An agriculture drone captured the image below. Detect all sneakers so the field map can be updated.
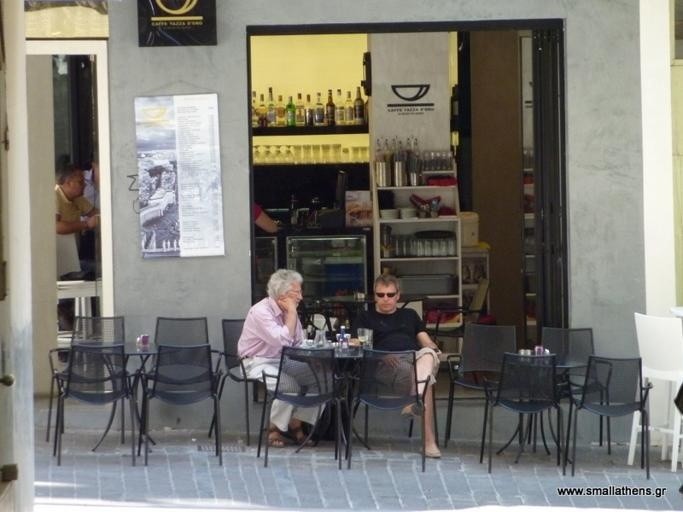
[420,446,441,458]
[401,402,426,421]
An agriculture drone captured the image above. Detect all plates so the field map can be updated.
[380,207,417,221]
[427,177,458,186]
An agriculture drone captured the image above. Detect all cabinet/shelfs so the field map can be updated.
[369,157,461,362]
[250,124,368,294]
[462,243,491,322]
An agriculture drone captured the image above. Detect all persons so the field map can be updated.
[252,199,278,235]
[53,162,100,282]
[345,273,444,459]
[78,160,99,261]
[237,267,329,448]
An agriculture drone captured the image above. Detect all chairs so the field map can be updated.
[424,278,491,358]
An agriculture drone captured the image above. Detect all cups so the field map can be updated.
[253,144,368,164]
[357,328,371,351]
[391,237,456,257]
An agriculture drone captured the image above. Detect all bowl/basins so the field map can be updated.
[301,333,365,348]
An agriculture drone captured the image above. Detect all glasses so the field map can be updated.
[375,293,397,298]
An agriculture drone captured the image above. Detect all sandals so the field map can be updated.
[282,424,312,445]
[266,427,285,447]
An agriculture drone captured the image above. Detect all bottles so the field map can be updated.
[372,137,423,189]
[421,151,454,171]
[251,85,364,127]
[336,325,349,357]
[287,199,299,224]
[313,329,328,349]
